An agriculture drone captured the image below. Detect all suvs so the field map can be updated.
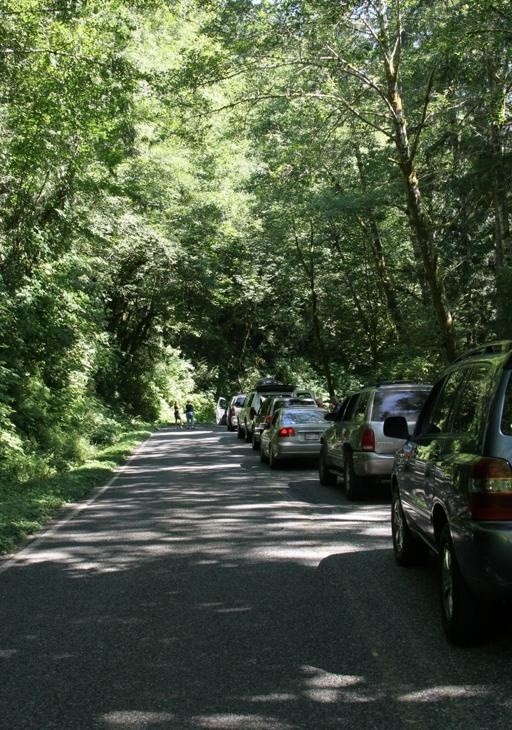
[382,339,511,649]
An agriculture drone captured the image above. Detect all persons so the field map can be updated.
[186,399,195,429]
[174,401,182,430]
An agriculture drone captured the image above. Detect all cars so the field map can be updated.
[250,397,319,451]
[258,405,336,471]
[318,379,434,502]
[215,384,316,444]
